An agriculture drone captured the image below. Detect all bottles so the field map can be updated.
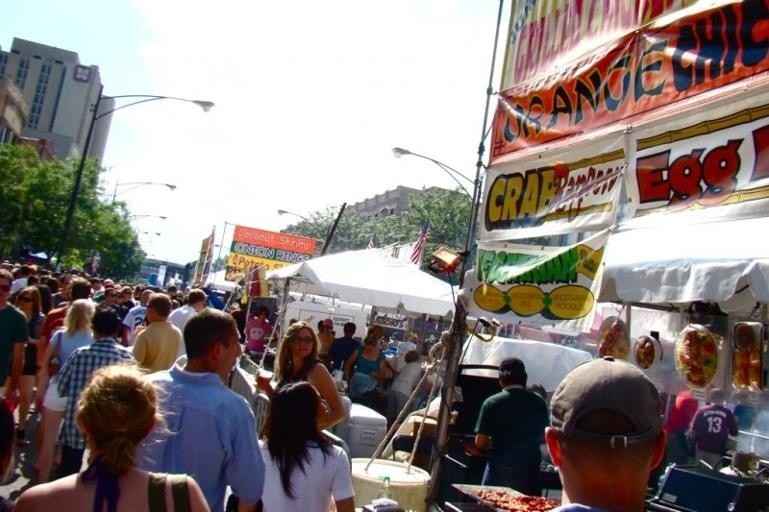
[378,477,390,500]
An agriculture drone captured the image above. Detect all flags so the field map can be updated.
[407,217,431,264]
[364,234,379,252]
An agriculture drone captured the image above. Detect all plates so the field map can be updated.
[597,316,719,390]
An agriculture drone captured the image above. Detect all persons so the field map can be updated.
[660,385,767,469]
[540,358,668,511]
[1,258,451,512]
[470,356,550,495]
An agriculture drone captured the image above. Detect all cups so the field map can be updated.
[255,370,273,393]
[335,370,343,382]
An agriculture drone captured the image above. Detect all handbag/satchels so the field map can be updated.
[225,491,264,512]
[47,331,62,374]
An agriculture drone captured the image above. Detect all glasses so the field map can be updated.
[16,296,34,303]
[292,334,314,344]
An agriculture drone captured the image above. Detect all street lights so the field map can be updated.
[392,144,483,289]
[57,87,217,281]
[276,209,333,253]
[111,179,177,238]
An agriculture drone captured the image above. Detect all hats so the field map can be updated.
[547,354,664,448]
[322,318,334,329]
